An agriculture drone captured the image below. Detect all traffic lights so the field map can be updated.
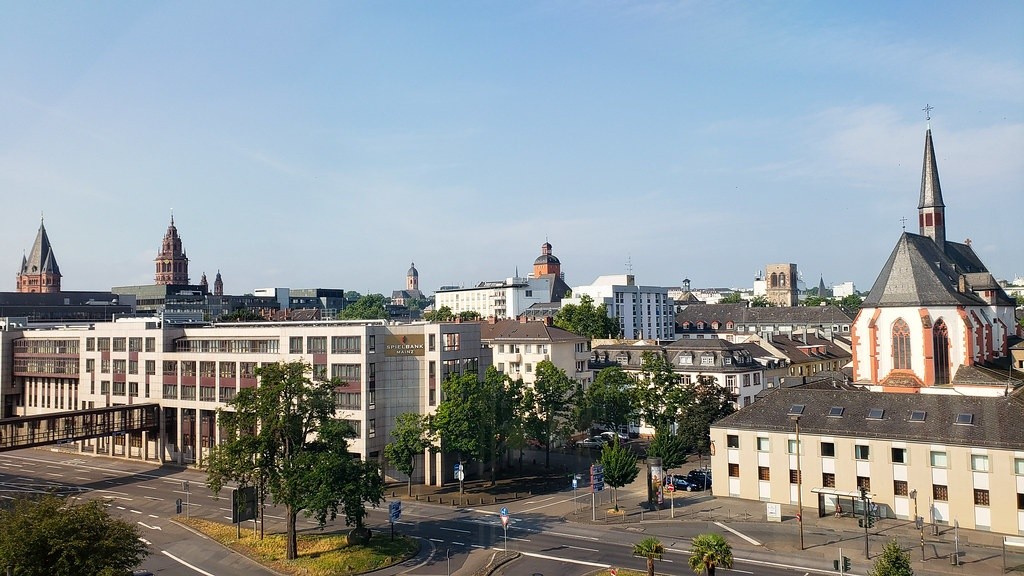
[860,487,867,500]
[833,560,839,571]
[843,557,851,572]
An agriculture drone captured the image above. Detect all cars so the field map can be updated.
[687,469,712,489]
[575,431,630,450]
[663,475,703,492]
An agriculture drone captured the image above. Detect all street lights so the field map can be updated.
[791,415,803,550]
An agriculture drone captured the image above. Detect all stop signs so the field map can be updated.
[796,514,800,520]
[667,483,674,492]
